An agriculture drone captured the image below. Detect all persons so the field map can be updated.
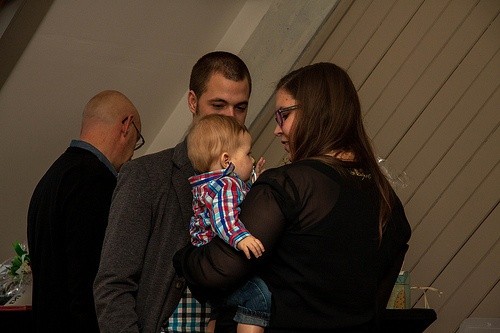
[172,62,411,332]
[28,89,145,333]
[94,52,261,333]
[188,113,269,333]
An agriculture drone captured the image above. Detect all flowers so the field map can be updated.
[0,241,31,287]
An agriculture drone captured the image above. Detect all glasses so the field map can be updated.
[122,117,145,150]
[275,105,299,128]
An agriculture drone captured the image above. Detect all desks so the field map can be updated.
[0,303,438,333]
